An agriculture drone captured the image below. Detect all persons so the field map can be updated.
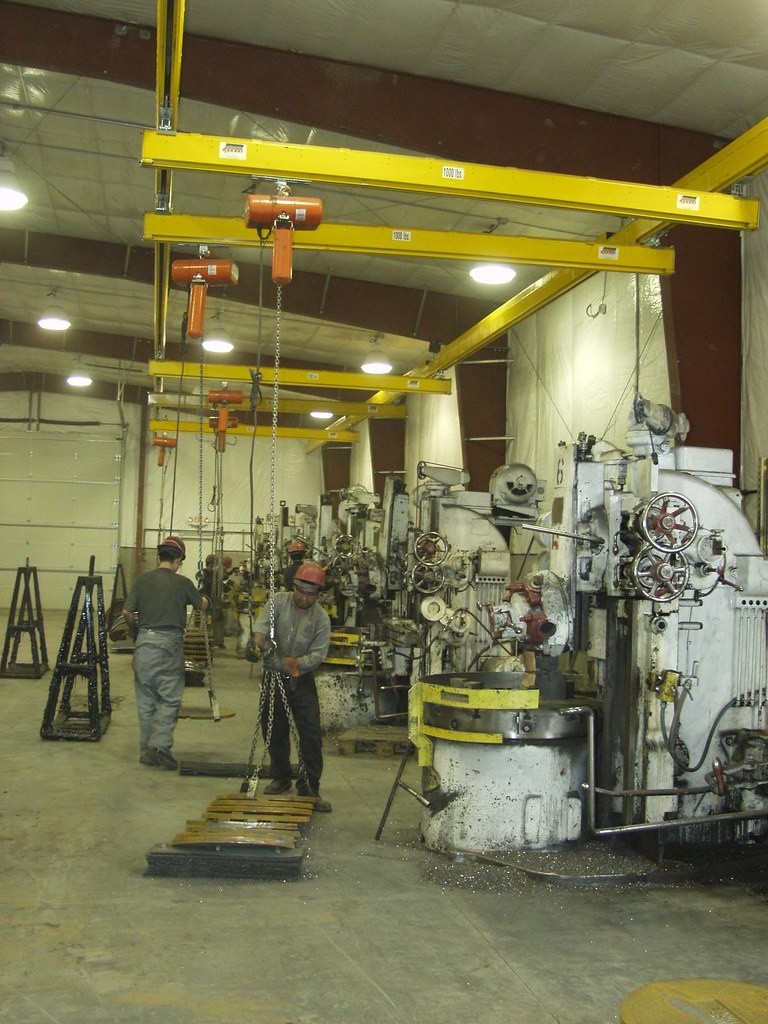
[244,562,331,812]
[283,540,308,592]
[122,535,209,771]
[221,556,237,636]
[202,553,212,597]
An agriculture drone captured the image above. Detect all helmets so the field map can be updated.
[156,536,187,562]
[205,554,215,563]
[287,541,307,553]
[223,557,232,565]
[292,563,325,590]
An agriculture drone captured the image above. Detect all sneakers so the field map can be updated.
[263,778,293,795]
[140,749,158,766]
[145,746,178,770]
[298,790,332,811]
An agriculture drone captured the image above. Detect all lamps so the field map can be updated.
[66,368,92,386]
[361,350,393,374]
[0,155,29,211]
[37,306,71,331]
[203,328,235,352]
